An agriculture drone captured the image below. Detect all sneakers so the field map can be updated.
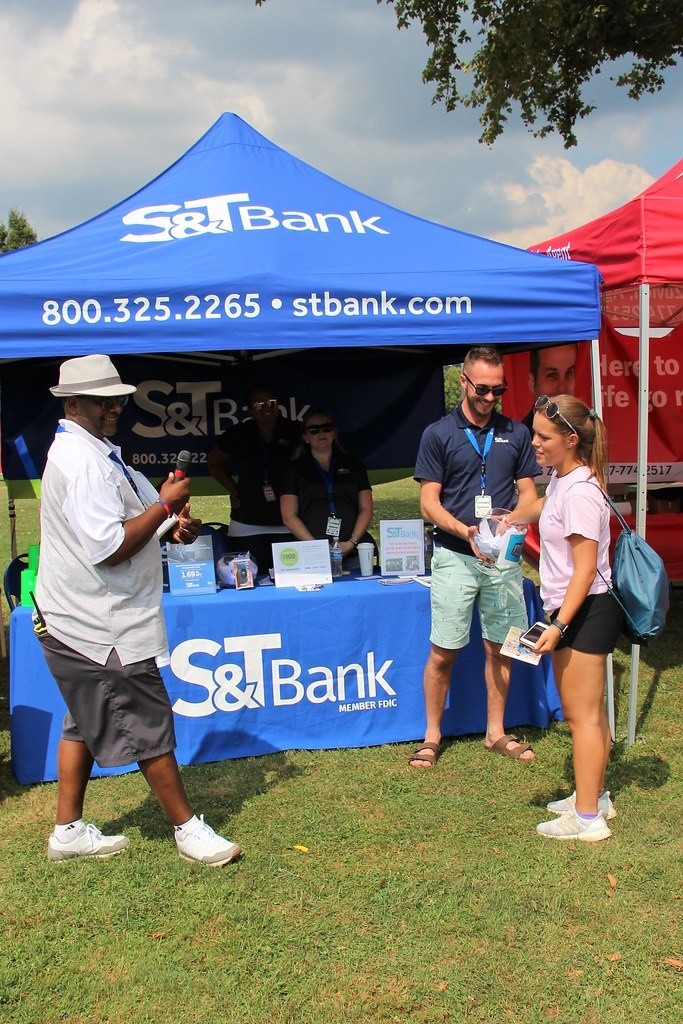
[536,803,612,842]
[175,814,242,866]
[48,824,130,864]
[547,789,616,820]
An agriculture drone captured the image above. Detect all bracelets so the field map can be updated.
[550,624,565,638]
[155,499,170,514]
[350,536,359,546]
[503,515,511,528]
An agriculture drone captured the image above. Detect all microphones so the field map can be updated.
[173,450,191,483]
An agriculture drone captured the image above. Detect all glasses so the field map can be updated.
[253,399,277,410]
[463,373,508,396]
[305,423,333,435]
[75,395,128,411]
[535,395,577,434]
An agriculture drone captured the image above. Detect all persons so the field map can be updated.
[209,378,309,575]
[280,408,379,571]
[407,348,544,767]
[32,353,241,867]
[520,340,578,442]
[494,394,626,841]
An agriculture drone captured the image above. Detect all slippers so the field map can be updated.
[408,742,440,768]
[484,733,535,761]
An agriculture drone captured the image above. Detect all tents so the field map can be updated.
[499,156,683,746]
[2,109,616,745]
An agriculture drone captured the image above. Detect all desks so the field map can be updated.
[8,582,563,784]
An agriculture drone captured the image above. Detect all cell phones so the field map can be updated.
[519,622,550,648]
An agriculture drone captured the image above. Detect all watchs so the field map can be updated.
[551,619,569,634]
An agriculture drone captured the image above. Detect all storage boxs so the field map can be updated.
[165,531,224,595]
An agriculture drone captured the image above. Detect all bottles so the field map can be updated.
[330,537,343,577]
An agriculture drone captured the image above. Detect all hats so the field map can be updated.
[50,354,137,396]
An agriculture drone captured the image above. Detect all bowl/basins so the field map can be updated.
[474,508,527,576]
[217,552,258,586]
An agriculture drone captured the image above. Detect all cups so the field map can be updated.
[357,543,375,576]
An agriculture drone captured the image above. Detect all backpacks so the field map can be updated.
[563,481,669,647]
